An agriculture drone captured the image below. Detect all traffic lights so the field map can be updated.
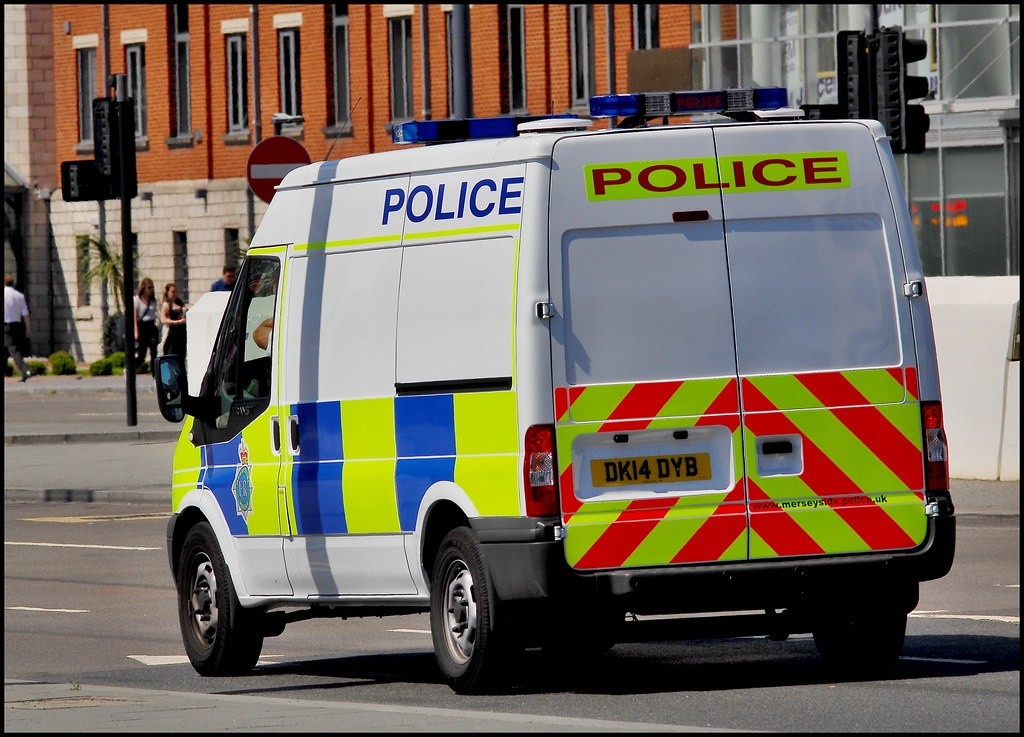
[90,98,134,177]
[866,24,931,158]
[799,103,841,120]
[836,29,874,121]
[60,159,138,201]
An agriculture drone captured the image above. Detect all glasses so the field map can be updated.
[145,286,154,289]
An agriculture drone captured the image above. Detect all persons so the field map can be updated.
[160,283,190,360]
[123,277,162,379]
[211,264,238,291]
[4,273,33,382]
[253,317,273,357]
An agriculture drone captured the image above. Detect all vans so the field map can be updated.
[151,85,958,696]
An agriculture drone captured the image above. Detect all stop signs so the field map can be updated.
[246,136,311,205]
